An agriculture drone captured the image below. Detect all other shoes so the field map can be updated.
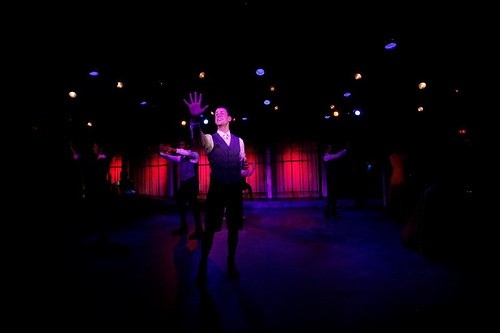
[197,259,207,273]
[227,254,240,277]
[172,227,188,236]
[188,230,203,240]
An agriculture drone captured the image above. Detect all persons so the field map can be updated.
[182,91,249,279]
[69,141,108,200]
[145,139,203,240]
[321,144,347,220]
